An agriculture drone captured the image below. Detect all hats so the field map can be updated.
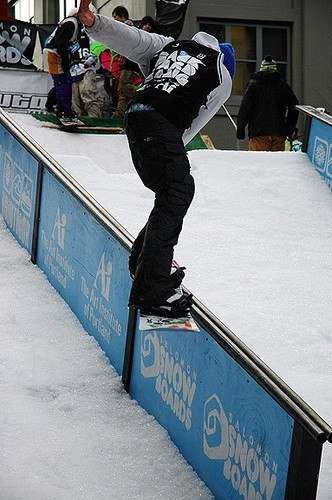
[219,43,235,81]
[259,55,277,72]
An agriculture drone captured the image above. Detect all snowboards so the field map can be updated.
[137,259,201,333]
[41,107,85,127]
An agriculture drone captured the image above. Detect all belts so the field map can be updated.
[125,103,155,114]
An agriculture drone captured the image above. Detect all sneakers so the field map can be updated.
[129,266,185,289]
[140,292,194,318]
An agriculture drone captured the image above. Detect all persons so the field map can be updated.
[235,56,299,151]
[76,7,234,319]
[285,123,303,151]
[43,3,153,128]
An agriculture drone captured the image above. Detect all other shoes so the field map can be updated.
[63,117,80,122]
